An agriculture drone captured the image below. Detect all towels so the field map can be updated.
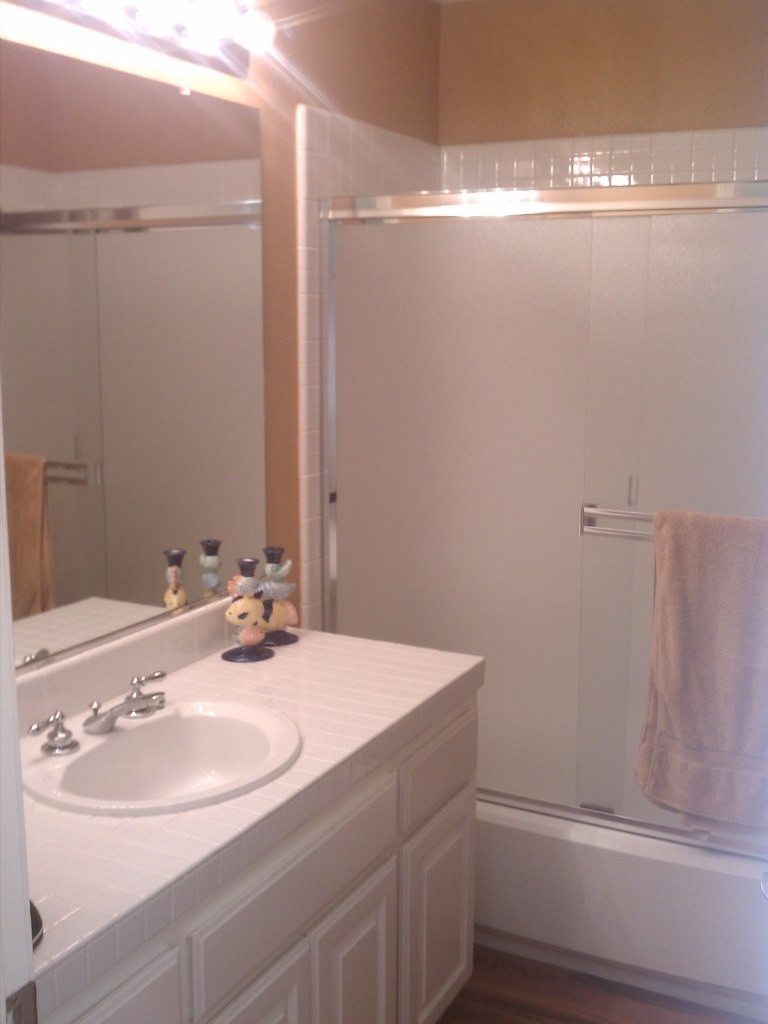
[5,449,58,622]
[632,505,768,834]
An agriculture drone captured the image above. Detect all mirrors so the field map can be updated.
[0,37,268,668]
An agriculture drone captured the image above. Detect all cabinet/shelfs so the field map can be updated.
[72,693,478,1024]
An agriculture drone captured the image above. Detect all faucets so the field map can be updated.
[83,692,166,736]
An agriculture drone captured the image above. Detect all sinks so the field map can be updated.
[21,698,300,818]
